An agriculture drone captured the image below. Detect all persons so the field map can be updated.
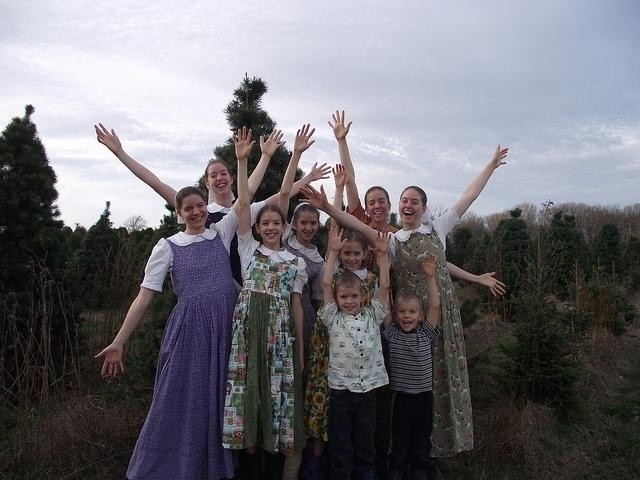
[317,227,388,479]
[222,128,308,480]
[94,124,332,290]
[303,164,388,480]
[278,124,345,480]
[298,144,509,479]
[376,255,441,479]
[328,110,401,233]
[94,131,288,480]
